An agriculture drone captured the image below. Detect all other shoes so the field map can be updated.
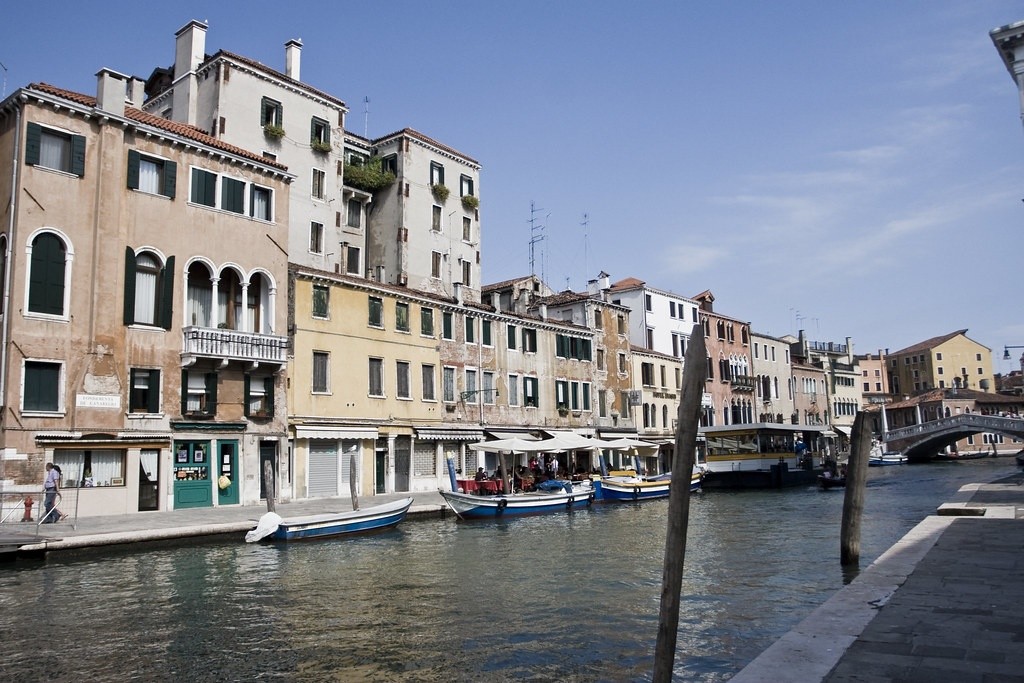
[42,521,52,523]
[54,515,61,523]
[60,514,68,520]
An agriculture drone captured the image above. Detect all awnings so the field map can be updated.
[545,431,583,437]
[33,429,171,443]
[415,429,486,441]
[295,424,379,440]
[489,432,538,440]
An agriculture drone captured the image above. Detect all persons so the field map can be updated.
[454,455,615,495]
[41,462,62,524]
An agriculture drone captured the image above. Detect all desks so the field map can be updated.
[456,478,511,496]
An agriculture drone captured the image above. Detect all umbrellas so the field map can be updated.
[468,437,657,494]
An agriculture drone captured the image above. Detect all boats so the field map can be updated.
[439,489,597,519]
[601,472,702,501]
[699,425,838,490]
[818,474,847,487]
[275,496,414,538]
[869,453,908,465]
